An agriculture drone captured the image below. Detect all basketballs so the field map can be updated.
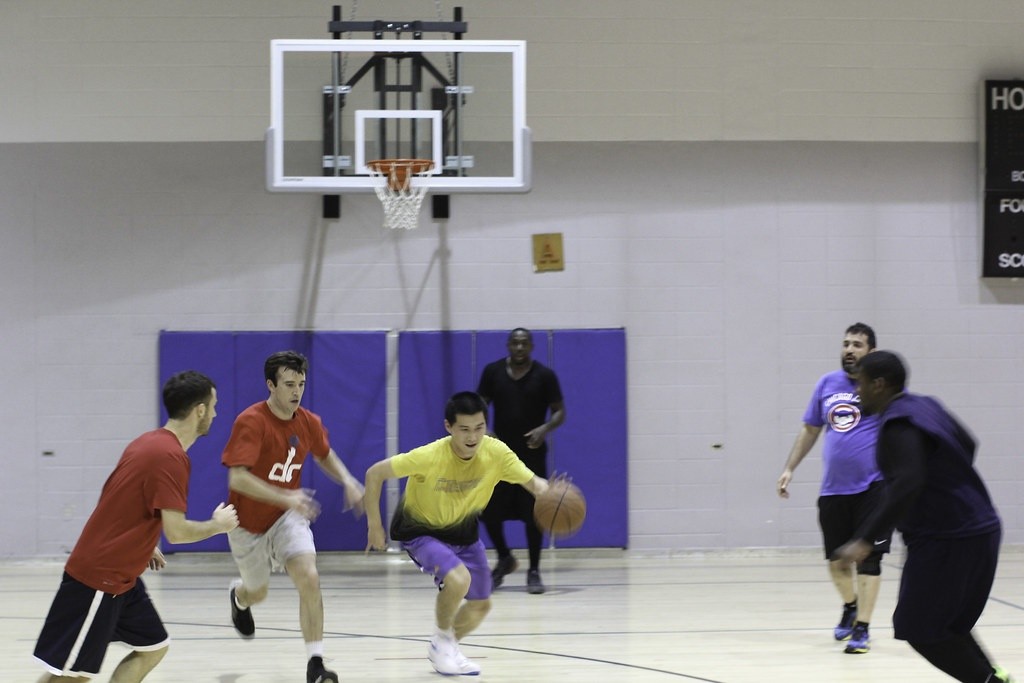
[530,482,586,536]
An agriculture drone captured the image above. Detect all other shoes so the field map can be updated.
[991,665,1016,683]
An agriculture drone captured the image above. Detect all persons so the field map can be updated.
[34,370,241,683]
[775,324,895,653]
[834,351,1011,683]
[364,390,570,676]
[222,351,362,683]
[477,328,566,594]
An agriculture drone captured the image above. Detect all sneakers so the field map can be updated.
[834,598,857,641]
[228,579,256,639]
[844,624,872,652]
[490,553,517,589]
[527,568,546,594]
[427,635,460,675]
[454,642,482,675]
[306,655,339,683]
